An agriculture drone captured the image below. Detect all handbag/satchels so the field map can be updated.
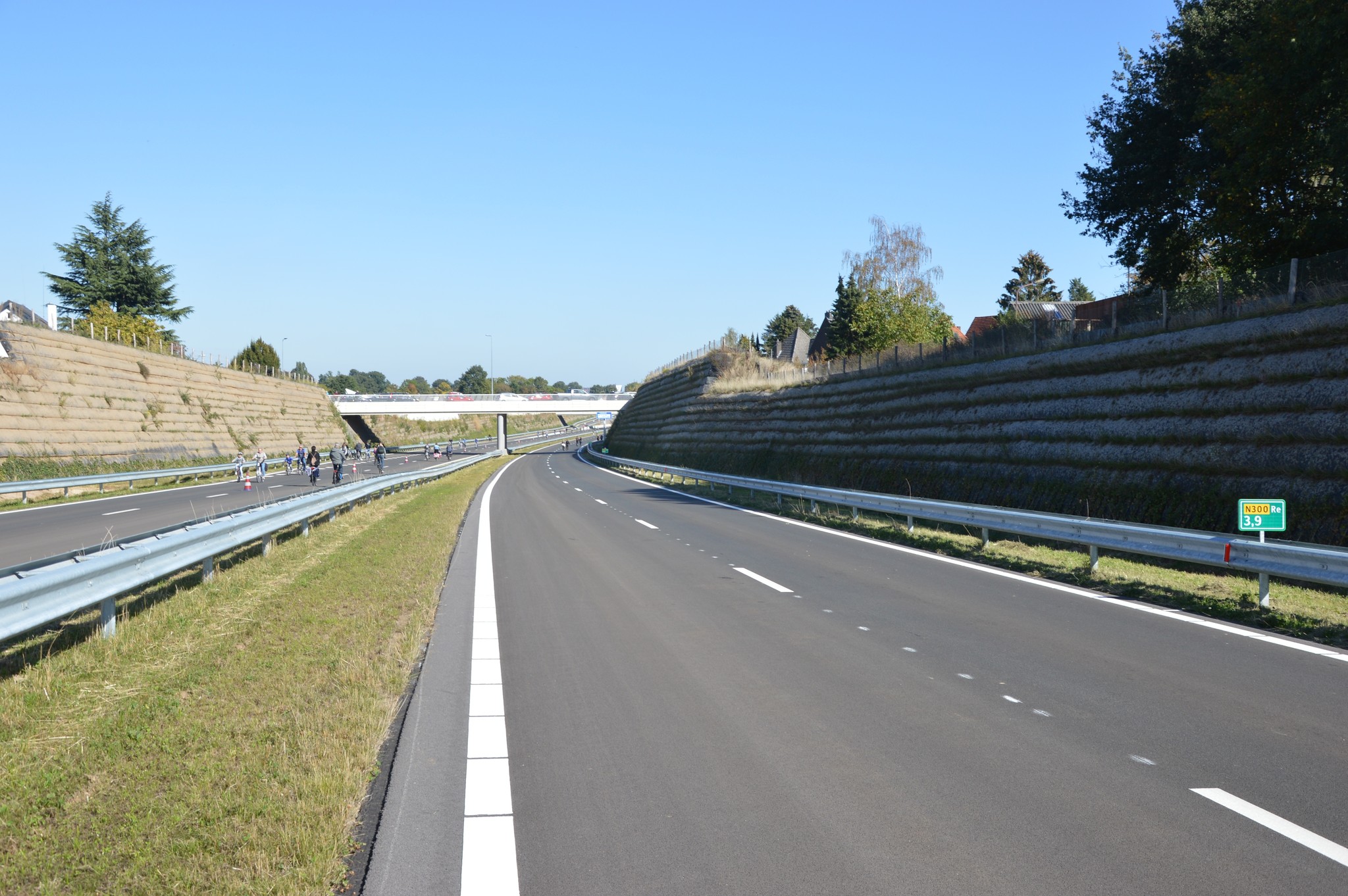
[265,463,268,471]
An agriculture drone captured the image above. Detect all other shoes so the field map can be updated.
[332,482,335,484]
[263,477,265,479]
[381,470,384,472]
[235,472,237,475]
[241,476,243,478]
[291,470,293,472]
[378,464,379,466]
[563,449,565,450]
[342,476,344,479]
[302,469,305,472]
[310,480,312,482]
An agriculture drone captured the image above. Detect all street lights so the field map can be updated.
[486,335,500,403]
[1015,279,1047,318]
[281,337,288,376]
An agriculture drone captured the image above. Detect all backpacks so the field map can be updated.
[311,452,317,466]
[378,446,384,454]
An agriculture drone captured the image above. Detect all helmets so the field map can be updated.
[238,452,242,455]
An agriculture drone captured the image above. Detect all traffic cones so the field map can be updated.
[243,472,254,492]
[484,444,487,448]
[405,455,409,463]
[352,461,359,474]
[464,447,468,453]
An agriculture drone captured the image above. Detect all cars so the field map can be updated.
[328,388,422,401]
[447,393,474,401]
[496,388,637,401]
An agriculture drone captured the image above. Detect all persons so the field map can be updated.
[562,439,566,451]
[294,446,307,472]
[446,444,453,458]
[433,443,441,454]
[231,452,245,479]
[592,429,603,441]
[575,436,579,446]
[565,439,570,451]
[537,424,588,439]
[251,447,267,479]
[424,443,431,459]
[284,454,294,471]
[448,437,453,446]
[489,434,493,441]
[330,439,387,480]
[458,438,462,446]
[307,446,320,483]
[462,438,466,448]
[579,437,582,446]
[475,438,478,446]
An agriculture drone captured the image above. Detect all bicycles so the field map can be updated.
[459,442,466,449]
[253,457,267,483]
[435,452,439,462]
[309,466,318,486]
[296,456,305,475]
[364,448,370,462]
[447,452,453,461]
[342,447,365,460]
[374,454,382,474]
[234,461,248,485]
[426,452,429,462]
[333,464,343,485]
[284,460,294,476]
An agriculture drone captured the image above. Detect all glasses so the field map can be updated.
[299,447,302,448]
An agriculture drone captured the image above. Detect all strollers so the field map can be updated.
[475,442,479,448]
